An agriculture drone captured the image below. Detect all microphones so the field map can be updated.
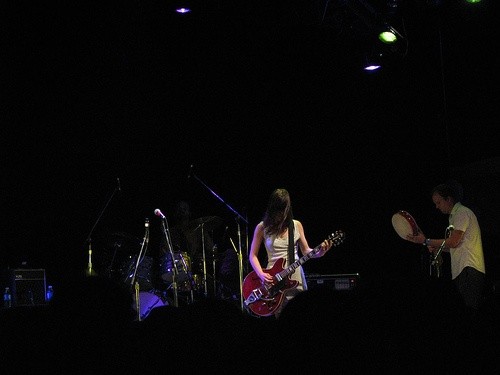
[185,164,194,180]
[117,178,122,190]
[445,224,456,233]
[154,208,166,219]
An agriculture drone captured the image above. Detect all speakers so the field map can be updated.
[12,268,47,306]
[304,272,361,293]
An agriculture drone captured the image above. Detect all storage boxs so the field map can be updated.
[9,269,47,307]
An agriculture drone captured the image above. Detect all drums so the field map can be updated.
[131,289,170,323]
[127,255,162,291]
[392,209,418,240]
[158,250,192,284]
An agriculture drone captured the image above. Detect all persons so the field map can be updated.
[160,201,214,258]
[407,184,486,294]
[248,190,333,316]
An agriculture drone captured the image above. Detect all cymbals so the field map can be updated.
[183,215,223,240]
[96,231,140,257]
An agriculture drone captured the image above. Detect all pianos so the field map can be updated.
[305,272,361,291]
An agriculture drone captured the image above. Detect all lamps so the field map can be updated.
[378,22,398,43]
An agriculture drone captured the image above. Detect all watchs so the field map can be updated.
[423,239,431,246]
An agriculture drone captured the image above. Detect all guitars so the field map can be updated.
[242,230,346,317]
